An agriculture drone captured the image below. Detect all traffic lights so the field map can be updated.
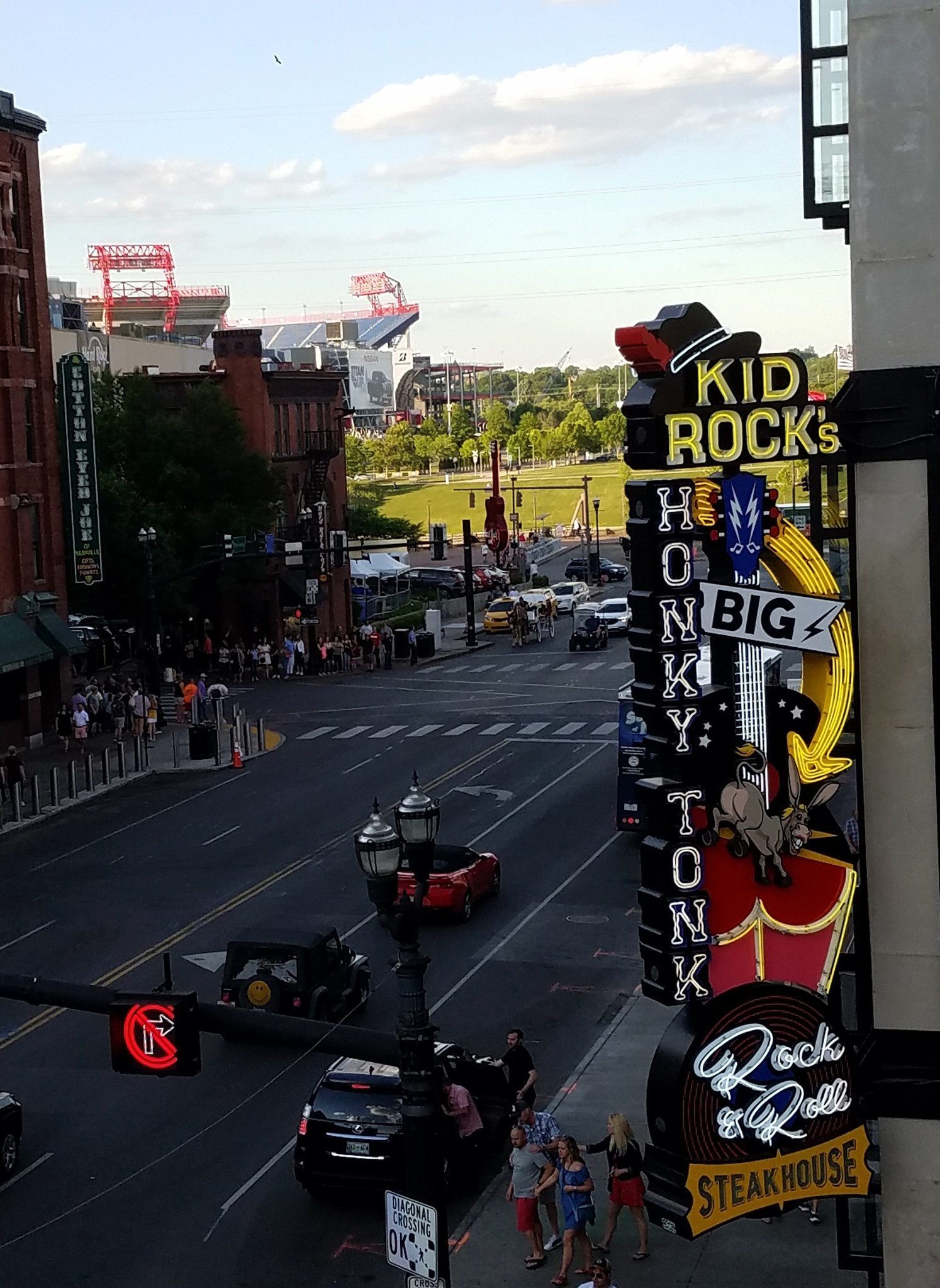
[295,608,305,619]
[516,491,523,508]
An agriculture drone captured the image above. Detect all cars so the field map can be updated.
[597,597,633,635]
[550,581,591,616]
[482,595,519,634]
[0,1091,23,1172]
[439,564,513,593]
[377,841,502,924]
[516,588,560,619]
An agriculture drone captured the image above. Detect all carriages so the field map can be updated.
[511,599,556,648]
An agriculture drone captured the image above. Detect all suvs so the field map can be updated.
[293,1043,507,1191]
[380,566,466,600]
[563,556,629,582]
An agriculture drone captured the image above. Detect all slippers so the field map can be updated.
[524,1254,548,1270]
[632,1251,651,1261]
[550,1274,568,1285]
[592,1244,610,1254]
[575,1266,590,1274]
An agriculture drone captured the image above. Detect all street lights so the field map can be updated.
[355,774,443,1285]
[511,477,518,558]
[138,527,158,623]
[593,499,604,587]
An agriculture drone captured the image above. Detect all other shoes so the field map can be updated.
[319,670,342,676]
[155,730,162,734]
[272,671,303,680]
[252,677,259,682]
[113,738,124,744]
[148,737,157,741]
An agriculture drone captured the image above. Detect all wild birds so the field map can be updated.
[274,54,282,65]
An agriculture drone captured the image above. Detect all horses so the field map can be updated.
[504,610,530,648]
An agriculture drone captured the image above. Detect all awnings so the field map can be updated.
[0,606,89,674]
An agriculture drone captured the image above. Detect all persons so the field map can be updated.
[56,670,229,754]
[184,615,393,684]
[506,1108,562,1270]
[577,1113,650,1261]
[534,1135,595,1287]
[439,1076,484,1196]
[519,597,531,612]
[530,561,538,575]
[486,1028,538,1125]
[408,625,420,668]
[494,586,502,600]
[0,745,26,806]
[578,1257,617,1288]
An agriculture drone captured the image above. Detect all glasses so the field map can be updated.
[610,1114,616,1119]
[512,1124,522,1130]
[590,1272,603,1279]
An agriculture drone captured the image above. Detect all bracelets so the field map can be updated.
[573,1186,577,1193]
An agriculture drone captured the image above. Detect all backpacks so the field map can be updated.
[211,688,221,699]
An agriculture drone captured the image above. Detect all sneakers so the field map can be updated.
[544,1234,563,1251]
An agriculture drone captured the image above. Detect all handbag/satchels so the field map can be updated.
[574,1204,596,1221]
[366,669,374,673]
[260,657,266,663]
[344,664,362,672]
[285,651,293,658]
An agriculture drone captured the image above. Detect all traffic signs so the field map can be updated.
[299,618,321,626]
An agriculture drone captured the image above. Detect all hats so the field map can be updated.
[509,1100,528,1117]
[8,745,16,753]
[200,673,208,677]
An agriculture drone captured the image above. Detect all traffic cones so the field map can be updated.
[229,739,246,769]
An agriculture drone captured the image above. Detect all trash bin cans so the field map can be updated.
[416,631,436,658]
[394,628,410,659]
[190,722,219,760]
